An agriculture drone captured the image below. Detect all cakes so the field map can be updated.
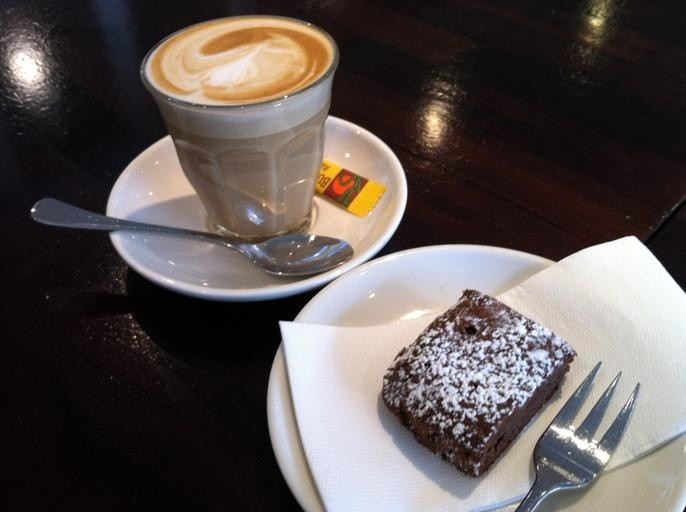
[382,288,576,478]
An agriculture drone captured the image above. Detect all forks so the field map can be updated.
[515,358,642,512]
[265,243,685,512]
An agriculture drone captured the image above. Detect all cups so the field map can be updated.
[141,13,341,245]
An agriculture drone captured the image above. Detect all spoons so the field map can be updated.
[29,196,355,278]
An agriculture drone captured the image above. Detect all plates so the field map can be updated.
[106,114,410,304]
